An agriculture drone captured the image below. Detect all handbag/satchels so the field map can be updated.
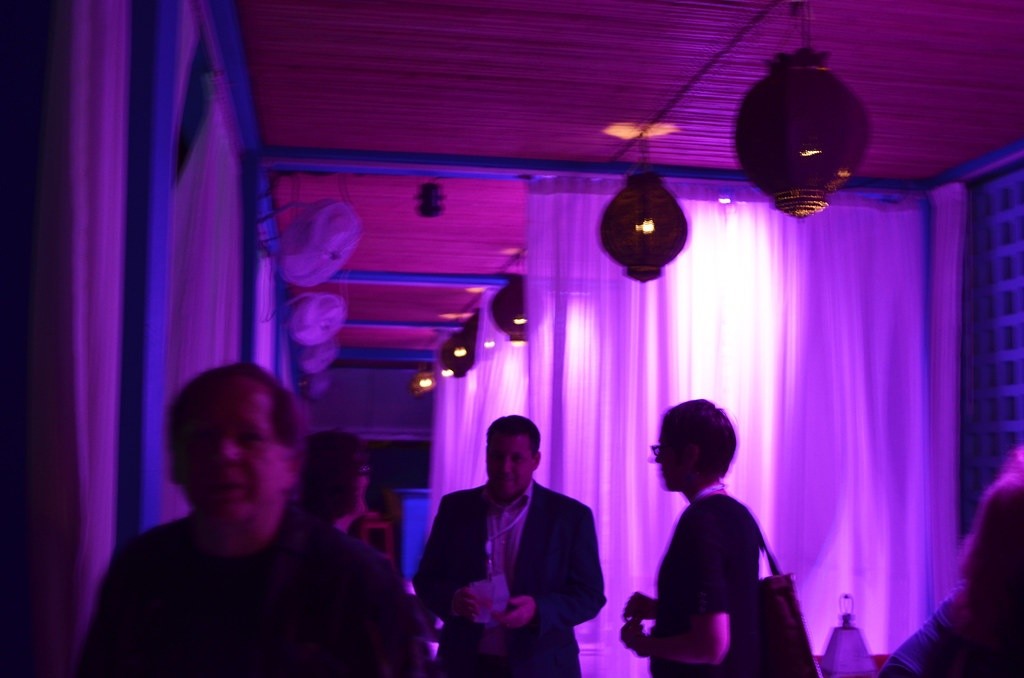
[741,500,823,678]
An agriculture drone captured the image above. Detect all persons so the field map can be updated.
[70,360,429,678]
[875,445,1024,678]
[411,414,608,678]
[619,398,774,678]
[295,429,380,532]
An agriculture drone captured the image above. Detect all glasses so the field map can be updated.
[359,465,369,474]
[652,444,667,457]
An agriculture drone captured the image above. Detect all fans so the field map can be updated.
[248,199,362,397]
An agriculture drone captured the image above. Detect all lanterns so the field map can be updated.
[438,330,475,377]
[411,369,437,399]
[595,171,687,282]
[488,282,528,342]
[728,48,868,220]
